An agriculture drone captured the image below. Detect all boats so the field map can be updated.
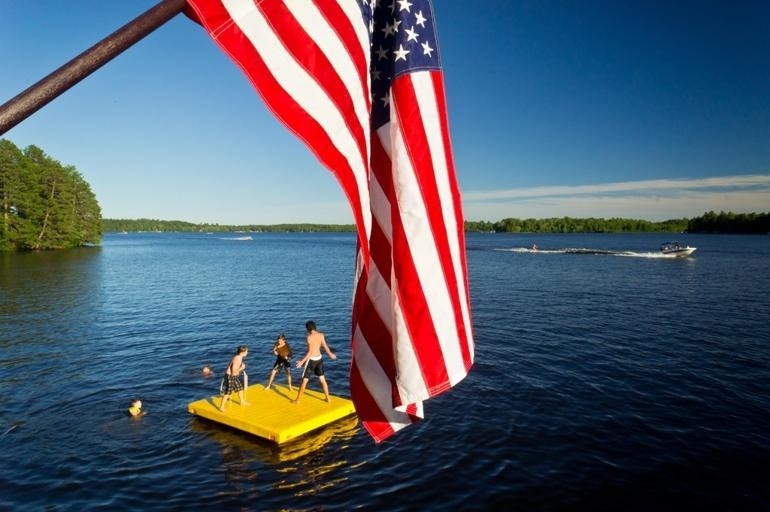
[661,242,697,258]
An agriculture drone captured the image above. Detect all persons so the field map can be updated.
[130,400,148,418]
[289,320,337,405]
[201,365,216,380]
[220,345,251,413]
[264,334,294,392]
[533,244,538,252]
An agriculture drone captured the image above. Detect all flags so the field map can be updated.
[178,0,476,445]
